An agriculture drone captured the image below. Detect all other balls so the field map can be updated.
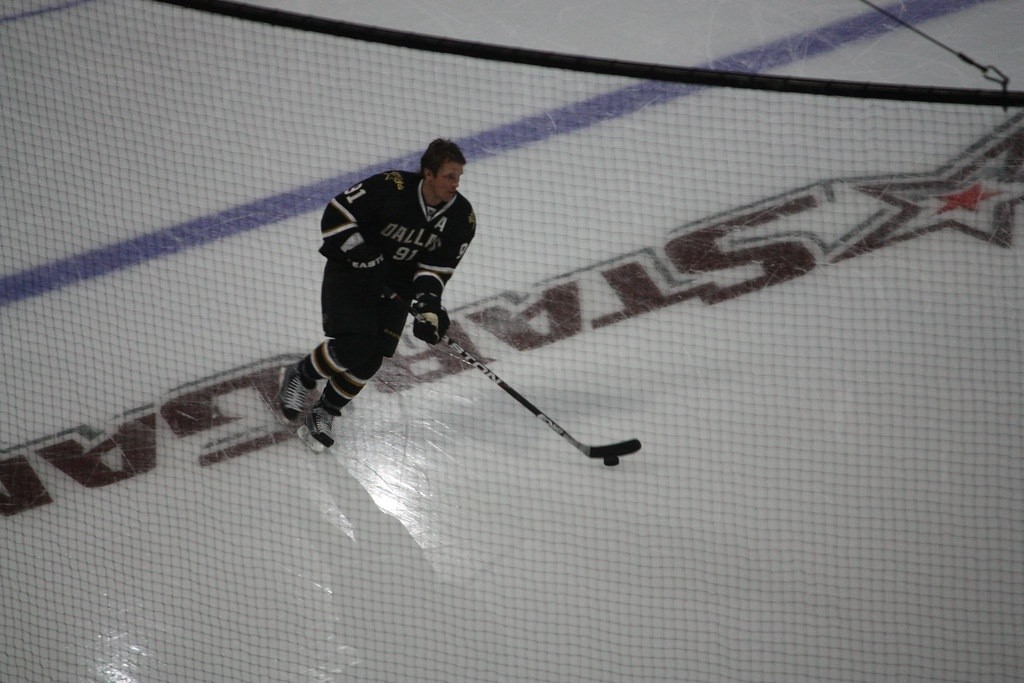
[603,456,619,466]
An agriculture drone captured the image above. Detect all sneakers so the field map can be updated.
[279,364,315,424]
[297,398,341,451]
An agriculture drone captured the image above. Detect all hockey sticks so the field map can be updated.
[384,289,644,461]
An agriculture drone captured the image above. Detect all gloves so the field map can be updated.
[412,293,451,345]
[343,241,393,287]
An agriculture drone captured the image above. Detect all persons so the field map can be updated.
[278,139,476,453]
